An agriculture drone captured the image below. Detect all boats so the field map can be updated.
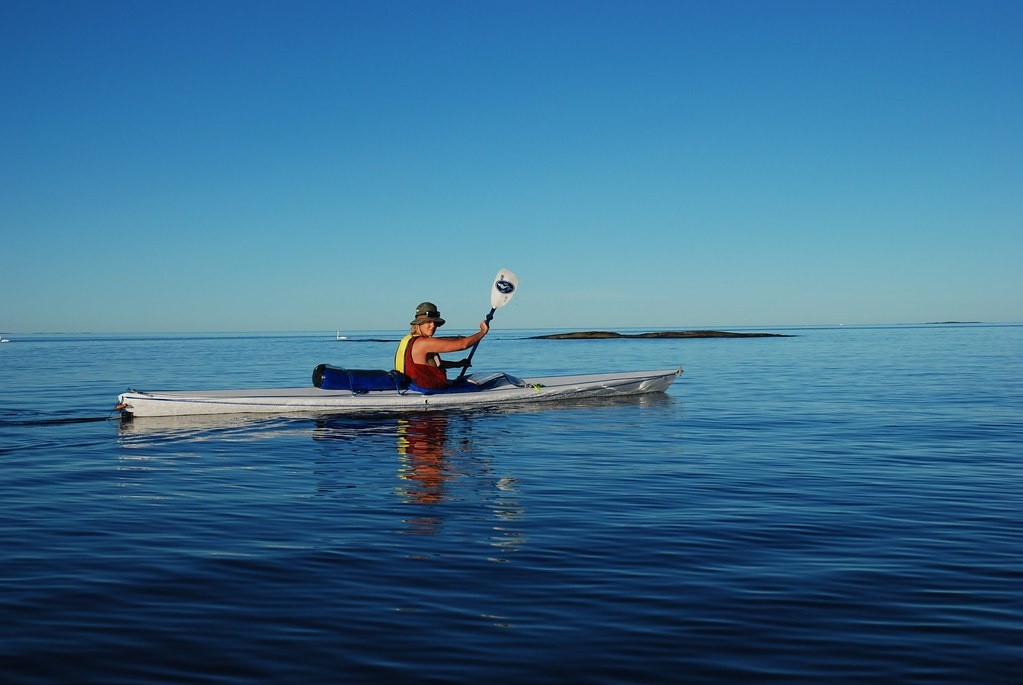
[116,366,685,418]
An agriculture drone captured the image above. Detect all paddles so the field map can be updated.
[459,268,518,377]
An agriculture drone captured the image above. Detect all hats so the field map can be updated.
[410,302,445,327]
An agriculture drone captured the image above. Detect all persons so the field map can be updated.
[395,302,489,394]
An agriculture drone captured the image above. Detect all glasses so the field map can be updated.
[415,311,440,318]
